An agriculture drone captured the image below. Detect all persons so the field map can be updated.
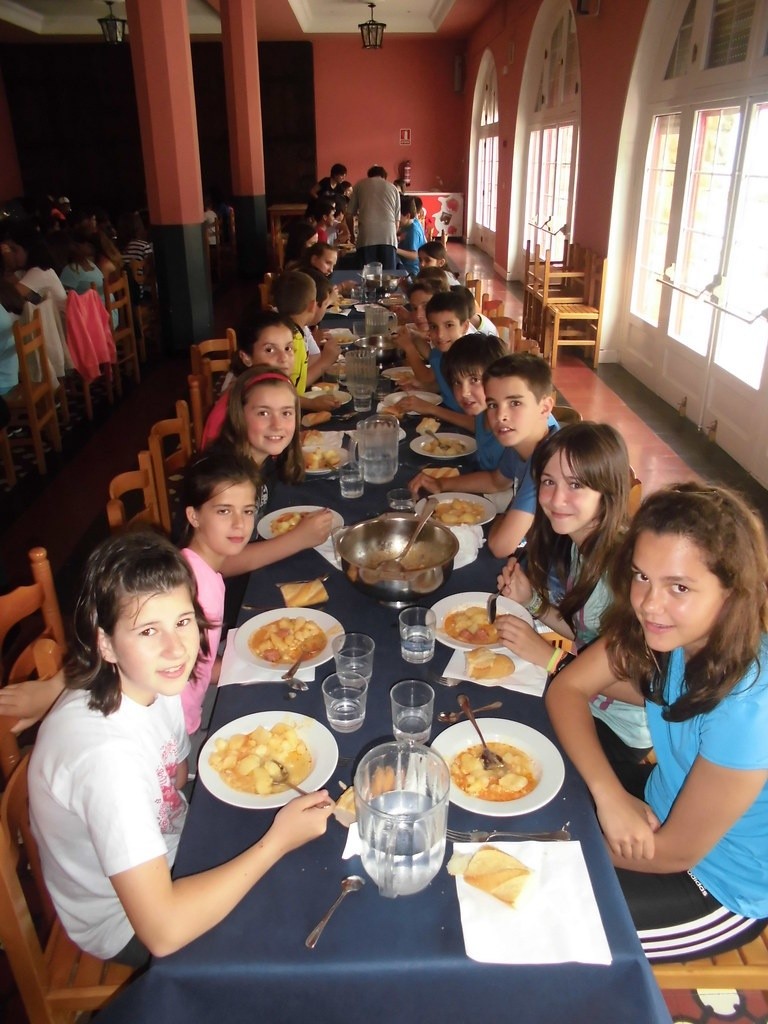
[0,452,261,775]
[283,194,359,292]
[0,196,158,436]
[409,352,560,557]
[204,198,232,249]
[496,420,656,765]
[28,539,334,969]
[390,249,561,472]
[201,267,340,465]
[547,481,767,964]
[311,163,428,271]
[218,364,335,636]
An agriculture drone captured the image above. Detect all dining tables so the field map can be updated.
[95,269,668,1024]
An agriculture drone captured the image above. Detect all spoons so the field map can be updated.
[281,636,323,680]
[425,429,452,450]
[436,701,503,723]
[487,549,526,626]
[458,695,504,770]
[263,760,331,810]
[305,875,365,949]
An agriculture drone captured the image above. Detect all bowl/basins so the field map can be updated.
[353,336,405,364]
[335,516,460,607]
[366,273,401,291]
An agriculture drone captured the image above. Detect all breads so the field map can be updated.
[280,578,329,607]
[303,430,325,445]
[338,784,368,815]
[422,467,459,478]
[463,845,530,907]
[381,406,405,418]
[416,418,441,435]
[463,647,515,681]
[331,305,339,313]
[302,411,332,427]
[313,382,340,390]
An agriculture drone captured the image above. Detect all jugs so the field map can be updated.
[336,346,380,399]
[348,414,399,485]
[364,306,398,336]
[353,739,451,899]
[364,261,382,288]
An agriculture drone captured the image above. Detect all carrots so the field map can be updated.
[211,721,309,795]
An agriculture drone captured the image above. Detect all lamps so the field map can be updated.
[358,3,386,48]
[98,1,126,44]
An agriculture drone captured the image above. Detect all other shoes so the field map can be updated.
[6,425,22,437]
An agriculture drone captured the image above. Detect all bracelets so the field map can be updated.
[341,282,344,290]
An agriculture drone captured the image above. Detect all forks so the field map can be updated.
[445,829,572,843]
[423,670,464,687]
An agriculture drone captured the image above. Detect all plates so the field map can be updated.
[430,717,566,817]
[410,433,477,460]
[329,298,359,309]
[384,391,443,415]
[381,365,431,381]
[233,607,346,673]
[319,337,360,347]
[425,592,534,652]
[303,391,352,404]
[198,711,339,809]
[301,446,350,475]
[414,492,496,527]
[257,506,343,540]
[377,298,409,306]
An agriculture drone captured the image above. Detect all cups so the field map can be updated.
[351,284,365,304]
[386,488,417,513]
[351,385,373,412]
[323,606,435,744]
[338,461,364,499]
[374,374,391,402]
[353,321,366,337]
[321,366,340,384]
[331,526,351,565]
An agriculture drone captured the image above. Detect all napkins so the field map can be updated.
[312,431,356,448]
[443,648,548,697]
[216,626,315,688]
[313,528,343,571]
[453,842,613,968]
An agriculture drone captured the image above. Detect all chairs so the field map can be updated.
[0,207,768,1024]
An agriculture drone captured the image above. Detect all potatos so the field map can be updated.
[258,616,320,662]
[433,498,486,523]
[453,607,498,644]
[424,438,463,454]
[276,512,303,533]
[306,446,339,469]
